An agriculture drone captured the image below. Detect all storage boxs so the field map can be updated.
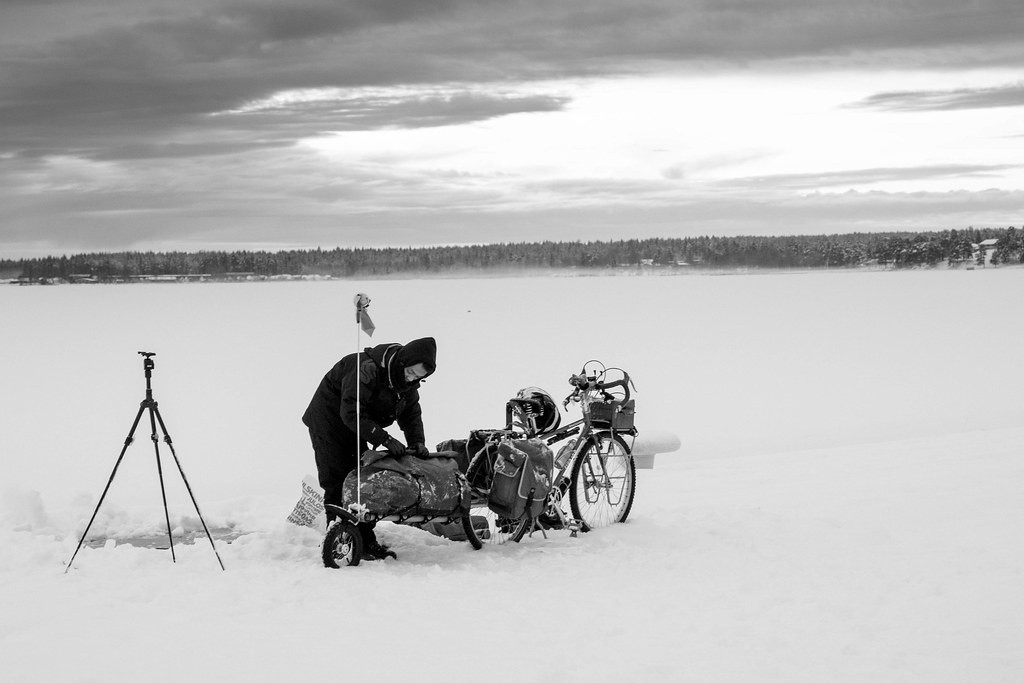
[589,401,634,429]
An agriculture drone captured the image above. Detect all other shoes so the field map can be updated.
[361,540,397,561]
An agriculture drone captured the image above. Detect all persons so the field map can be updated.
[302,336,437,561]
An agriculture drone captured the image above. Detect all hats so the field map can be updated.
[398,337,436,387]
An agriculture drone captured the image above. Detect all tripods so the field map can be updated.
[66,352,225,573]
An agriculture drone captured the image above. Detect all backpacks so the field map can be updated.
[436,428,554,522]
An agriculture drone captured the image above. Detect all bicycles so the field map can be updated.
[461,358,640,553]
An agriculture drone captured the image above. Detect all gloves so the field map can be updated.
[386,438,406,462]
[409,442,430,460]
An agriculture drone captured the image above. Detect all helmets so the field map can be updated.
[506,386,561,434]
